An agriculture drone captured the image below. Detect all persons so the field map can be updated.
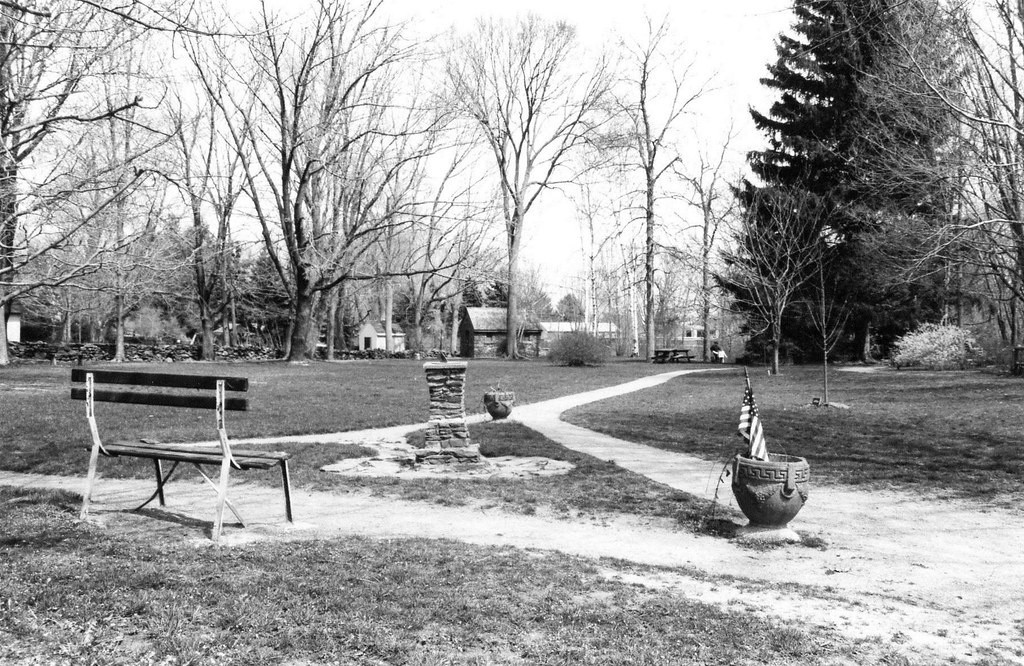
[709,341,721,362]
[713,349,728,363]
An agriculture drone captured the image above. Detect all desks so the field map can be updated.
[655,348,690,363]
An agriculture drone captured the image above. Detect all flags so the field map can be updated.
[737,385,772,464]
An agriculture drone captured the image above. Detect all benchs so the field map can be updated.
[70,367,295,542]
[652,355,669,363]
[672,355,695,363]
